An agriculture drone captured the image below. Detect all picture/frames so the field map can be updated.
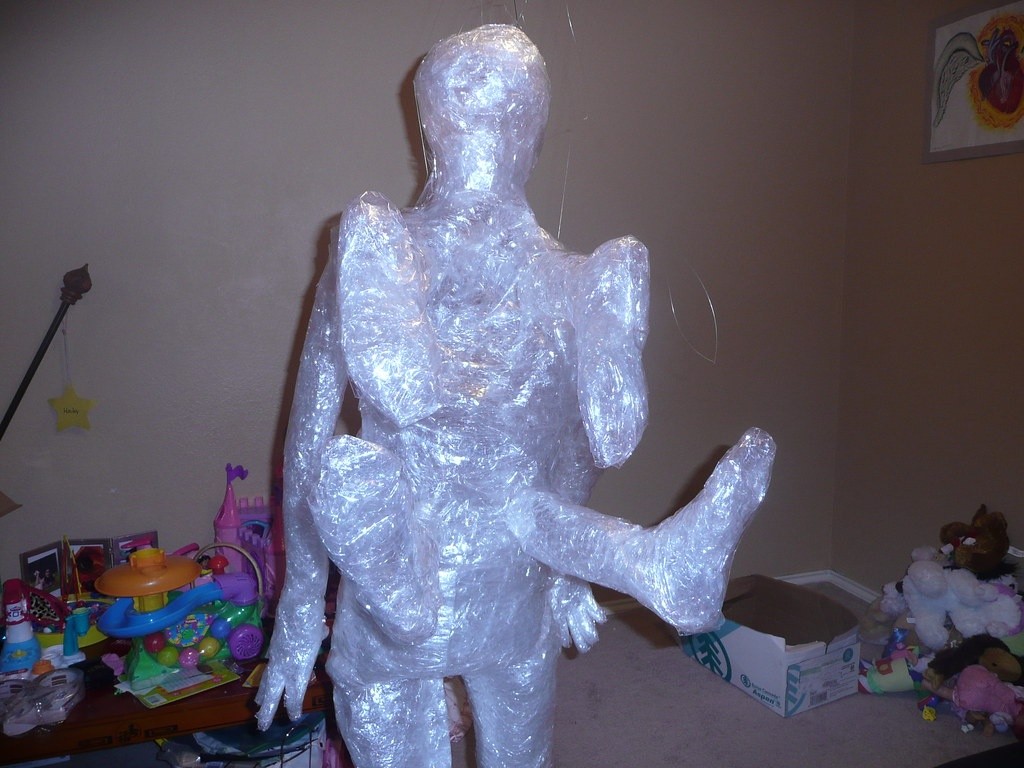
[60,538,112,601]
[112,530,158,566]
[19,540,62,597]
[919,0,1024,164]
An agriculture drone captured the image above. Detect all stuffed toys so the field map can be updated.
[855,502,1024,739]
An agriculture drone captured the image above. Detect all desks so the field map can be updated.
[0,634,331,768]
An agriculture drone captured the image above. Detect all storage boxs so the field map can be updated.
[672,573,861,718]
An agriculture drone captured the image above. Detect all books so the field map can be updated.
[118,660,241,709]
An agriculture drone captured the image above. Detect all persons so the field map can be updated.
[77,555,99,586]
[32,566,57,591]
